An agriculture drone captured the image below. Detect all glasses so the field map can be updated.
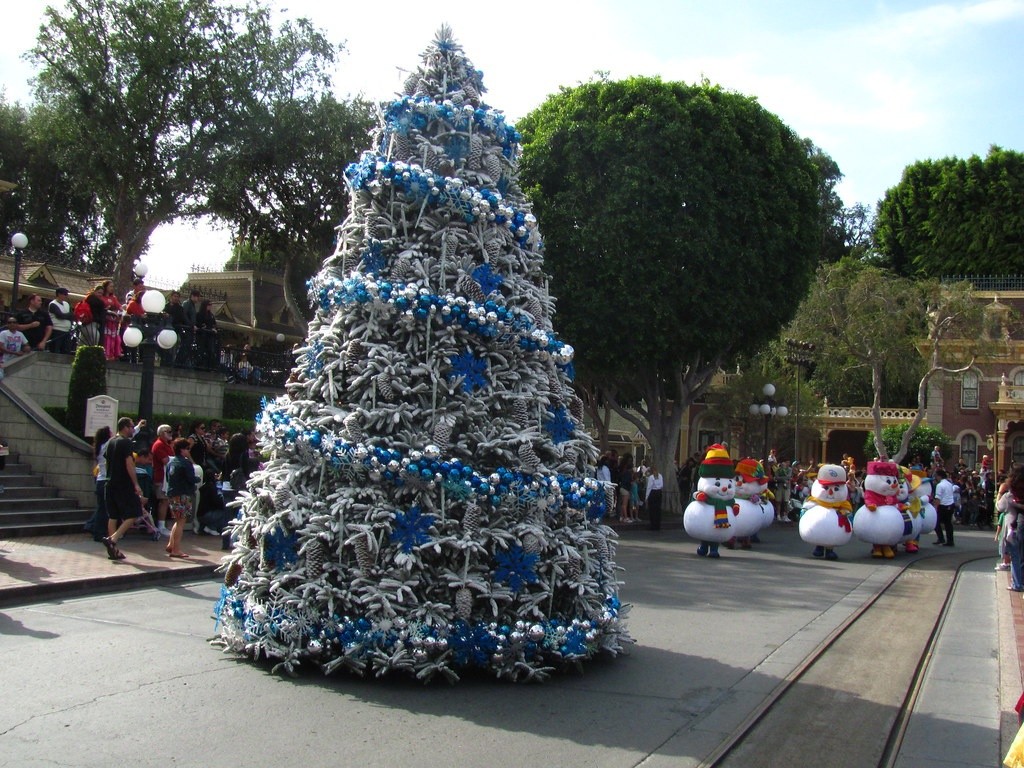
[165,431,172,433]
[183,447,190,450]
[198,427,205,430]
[127,425,135,429]
[214,426,221,427]
[8,321,16,324]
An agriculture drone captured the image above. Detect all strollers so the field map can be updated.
[121,467,162,543]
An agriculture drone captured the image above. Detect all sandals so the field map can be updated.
[102,536,126,559]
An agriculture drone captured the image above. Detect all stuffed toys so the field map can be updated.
[853,459,937,557]
[734,458,775,550]
[799,464,852,560]
[683,442,740,559]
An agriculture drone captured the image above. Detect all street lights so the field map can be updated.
[9,231,29,313]
[780,338,818,463]
[121,288,180,447]
[748,383,789,474]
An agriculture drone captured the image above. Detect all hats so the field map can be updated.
[56,287,69,293]
[192,289,204,297]
[134,278,143,285]
[792,461,798,466]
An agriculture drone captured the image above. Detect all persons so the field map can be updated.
[598,445,996,548]
[993,463,1024,592]
[0,279,306,388]
[95,417,262,560]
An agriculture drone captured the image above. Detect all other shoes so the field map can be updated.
[1006,586,1024,592]
[933,539,945,544]
[620,516,642,524]
[192,527,199,534]
[777,515,791,522]
[166,545,190,557]
[204,526,220,536]
[159,527,170,536]
[943,543,955,546]
[994,563,1009,571]
